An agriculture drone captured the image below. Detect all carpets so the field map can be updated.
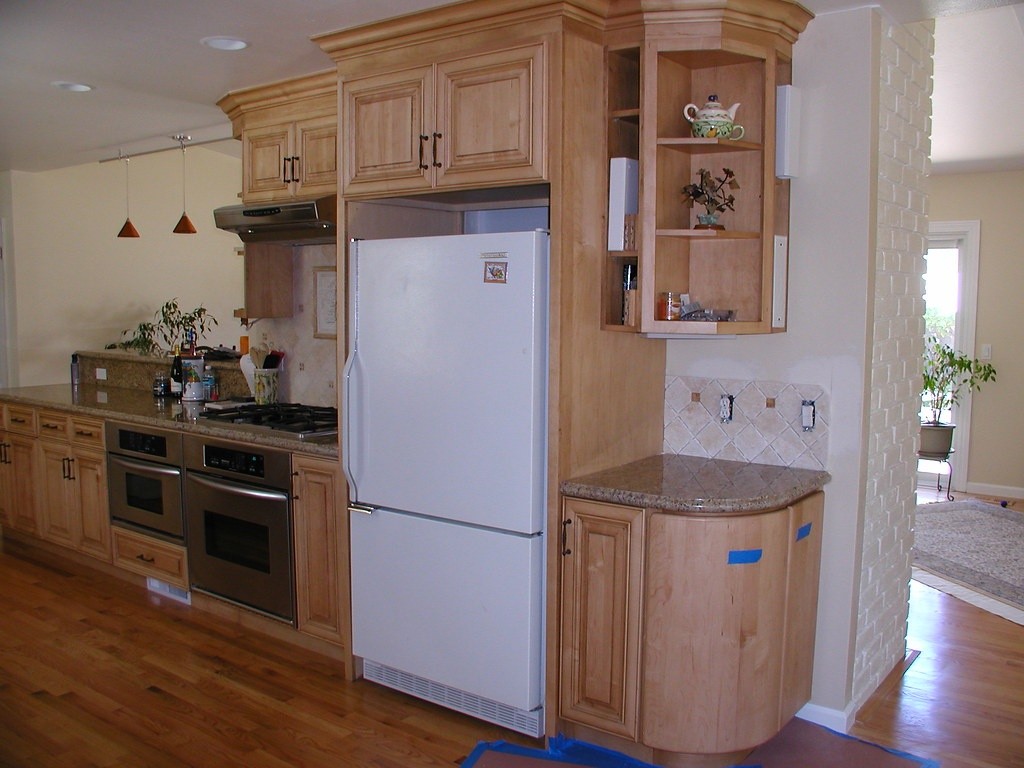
[910,498,1024,626]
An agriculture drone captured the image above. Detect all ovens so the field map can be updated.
[183,428,298,632]
[104,418,187,547]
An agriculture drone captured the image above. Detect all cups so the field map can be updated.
[253,368,279,405]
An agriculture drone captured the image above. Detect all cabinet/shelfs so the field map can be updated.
[0,400,347,678]
[308,0,668,745]
[595,0,816,341]
[560,454,832,768]
[214,65,337,202]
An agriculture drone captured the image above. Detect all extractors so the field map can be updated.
[214,200,336,244]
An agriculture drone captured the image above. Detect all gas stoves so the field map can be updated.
[194,395,338,438]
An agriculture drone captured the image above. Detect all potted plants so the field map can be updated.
[680,159,742,225]
[919,335,999,456]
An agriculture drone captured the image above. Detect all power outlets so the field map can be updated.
[718,393,734,424]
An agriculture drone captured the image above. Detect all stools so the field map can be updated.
[917,446,957,504]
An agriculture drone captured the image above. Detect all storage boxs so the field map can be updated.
[608,155,639,252]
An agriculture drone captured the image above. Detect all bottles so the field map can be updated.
[203,367,215,402]
[153,371,170,396]
[71,354,80,385]
[621,264,635,325]
[169,344,183,399]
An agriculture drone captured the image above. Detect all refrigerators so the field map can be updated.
[339,227,553,741]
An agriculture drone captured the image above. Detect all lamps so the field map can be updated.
[113,148,142,239]
[168,134,199,236]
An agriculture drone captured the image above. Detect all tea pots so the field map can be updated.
[684,94,745,140]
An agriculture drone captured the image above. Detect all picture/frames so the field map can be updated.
[312,265,337,340]
